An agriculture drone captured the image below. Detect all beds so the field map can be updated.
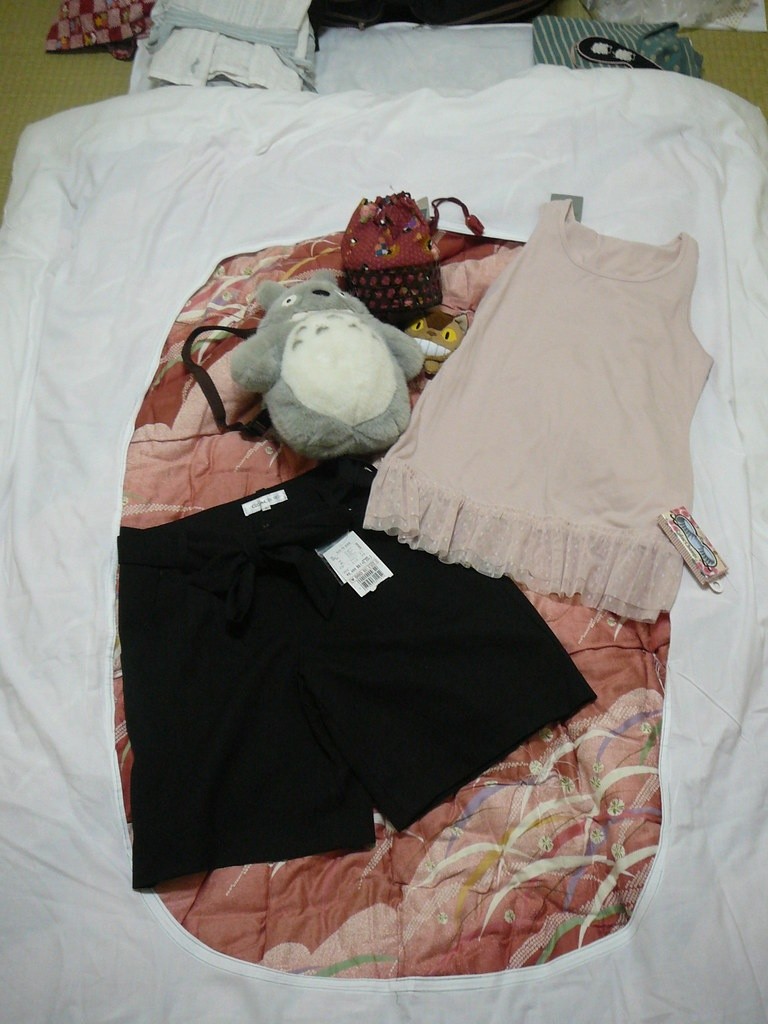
[0,67,768,1024]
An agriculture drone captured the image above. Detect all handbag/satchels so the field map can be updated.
[341,190,443,314]
